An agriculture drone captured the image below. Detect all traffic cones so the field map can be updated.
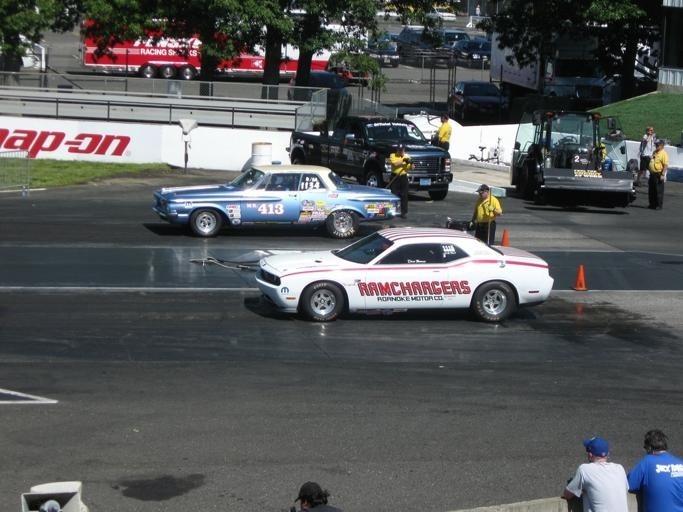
[502,229,510,247]
[570,263,590,291]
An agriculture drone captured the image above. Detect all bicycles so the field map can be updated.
[466,138,509,166]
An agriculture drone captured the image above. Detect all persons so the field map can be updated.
[390,145,414,220]
[267,175,278,191]
[585,136,612,171]
[471,184,504,244]
[437,114,453,151]
[629,428,681,510]
[294,481,342,511]
[648,135,666,210]
[641,127,654,180]
[564,437,629,512]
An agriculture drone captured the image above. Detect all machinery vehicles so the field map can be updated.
[511,110,636,209]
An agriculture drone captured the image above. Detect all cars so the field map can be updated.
[154,162,401,238]
[447,81,509,124]
[366,26,492,68]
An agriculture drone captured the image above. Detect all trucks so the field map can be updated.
[79,16,355,80]
[491,29,604,104]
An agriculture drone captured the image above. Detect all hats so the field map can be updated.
[475,184,490,192]
[582,436,610,459]
[295,481,324,503]
[654,139,665,145]
[396,142,406,150]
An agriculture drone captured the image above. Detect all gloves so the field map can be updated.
[407,160,414,166]
[403,157,409,163]
[469,220,475,229]
[487,205,496,213]
[659,173,665,182]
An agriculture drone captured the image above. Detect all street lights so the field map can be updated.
[17,33,46,89]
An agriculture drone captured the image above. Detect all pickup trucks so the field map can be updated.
[288,116,453,201]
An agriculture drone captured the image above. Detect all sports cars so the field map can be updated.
[254,226,553,321]
